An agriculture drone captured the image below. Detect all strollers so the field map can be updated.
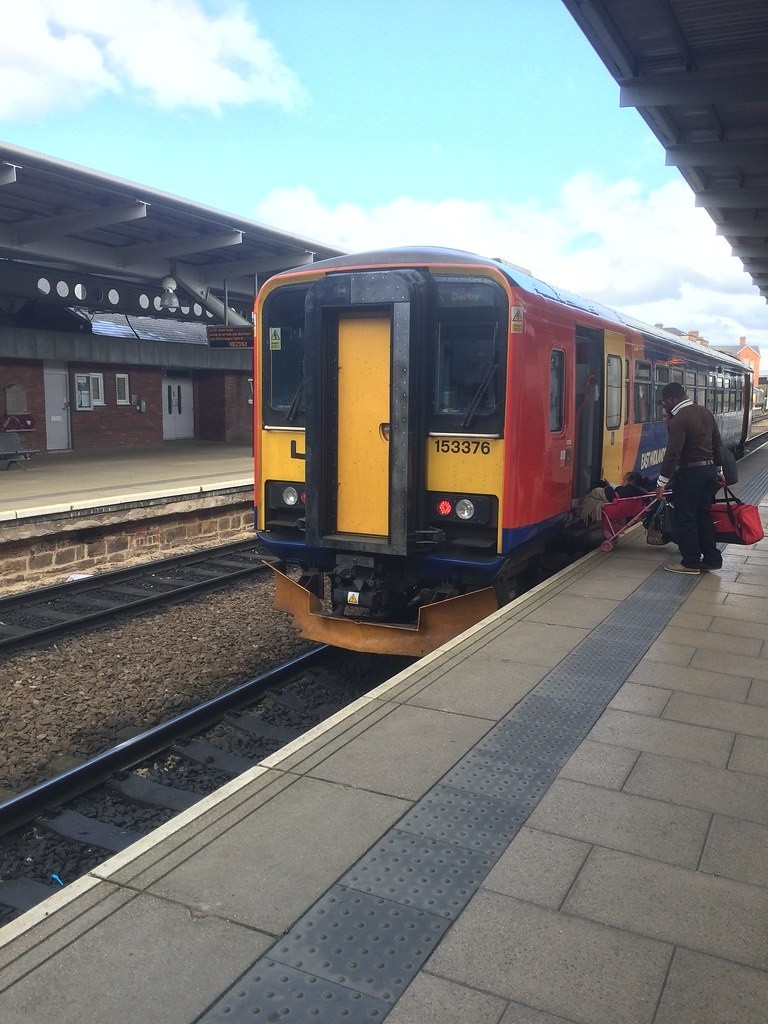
[573,489,676,553]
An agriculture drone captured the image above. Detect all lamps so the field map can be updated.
[160,277,181,309]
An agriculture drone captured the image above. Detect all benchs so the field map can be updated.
[0,433,41,471]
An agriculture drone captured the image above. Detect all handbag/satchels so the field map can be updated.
[710,486,764,545]
[643,499,674,545]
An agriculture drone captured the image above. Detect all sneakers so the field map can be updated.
[664,564,700,574]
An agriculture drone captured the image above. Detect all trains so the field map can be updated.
[250,244,757,655]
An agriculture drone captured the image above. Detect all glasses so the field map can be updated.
[661,395,672,405]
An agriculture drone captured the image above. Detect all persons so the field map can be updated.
[654,382,739,575]
[585,472,649,495]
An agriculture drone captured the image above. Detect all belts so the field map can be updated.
[688,460,714,467]
